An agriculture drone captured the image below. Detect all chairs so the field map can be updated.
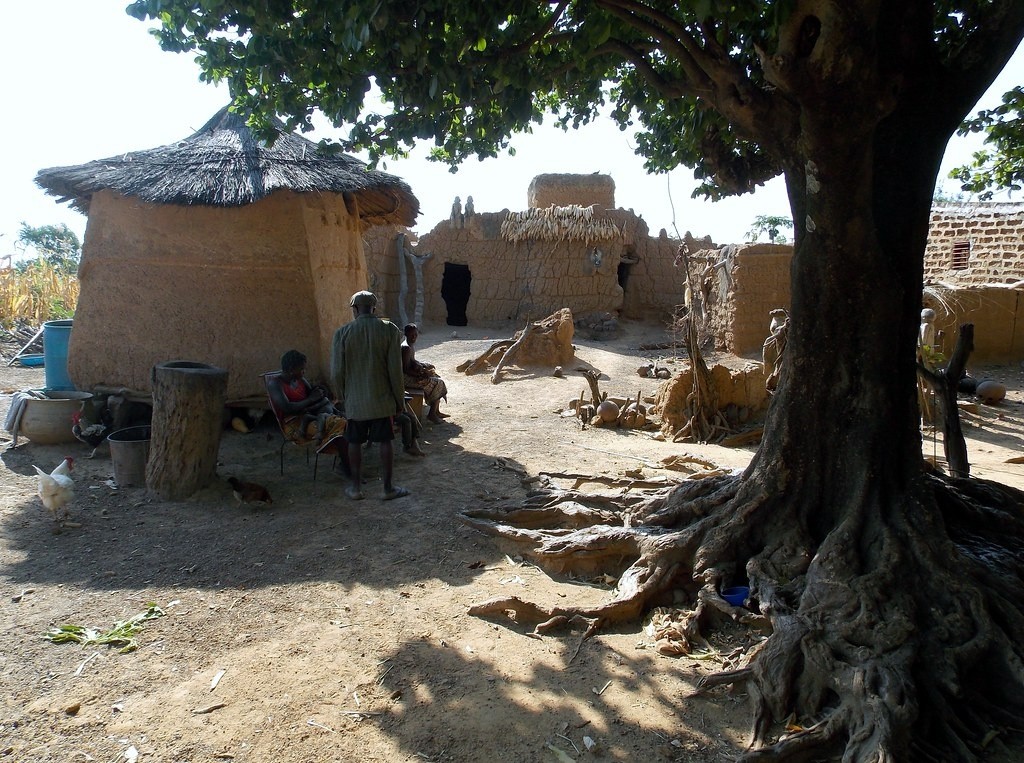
[259,371,347,481]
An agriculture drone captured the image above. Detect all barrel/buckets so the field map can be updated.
[107,425,150,487]
[43,319,74,390]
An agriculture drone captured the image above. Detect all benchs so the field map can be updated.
[403,388,424,422]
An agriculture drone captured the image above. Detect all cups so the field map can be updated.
[721,586,749,607]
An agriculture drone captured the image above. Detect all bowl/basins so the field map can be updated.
[20,390,94,445]
[16,353,44,366]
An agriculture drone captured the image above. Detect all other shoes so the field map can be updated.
[437,412,450,418]
[380,486,411,500]
[427,414,442,424]
[412,440,426,457]
[403,447,419,457]
[345,486,364,500]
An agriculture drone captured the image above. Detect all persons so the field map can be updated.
[330,291,411,500]
[394,413,424,456]
[400,323,451,425]
[267,350,366,485]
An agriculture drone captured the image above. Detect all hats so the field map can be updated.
[350,291,378,307]
